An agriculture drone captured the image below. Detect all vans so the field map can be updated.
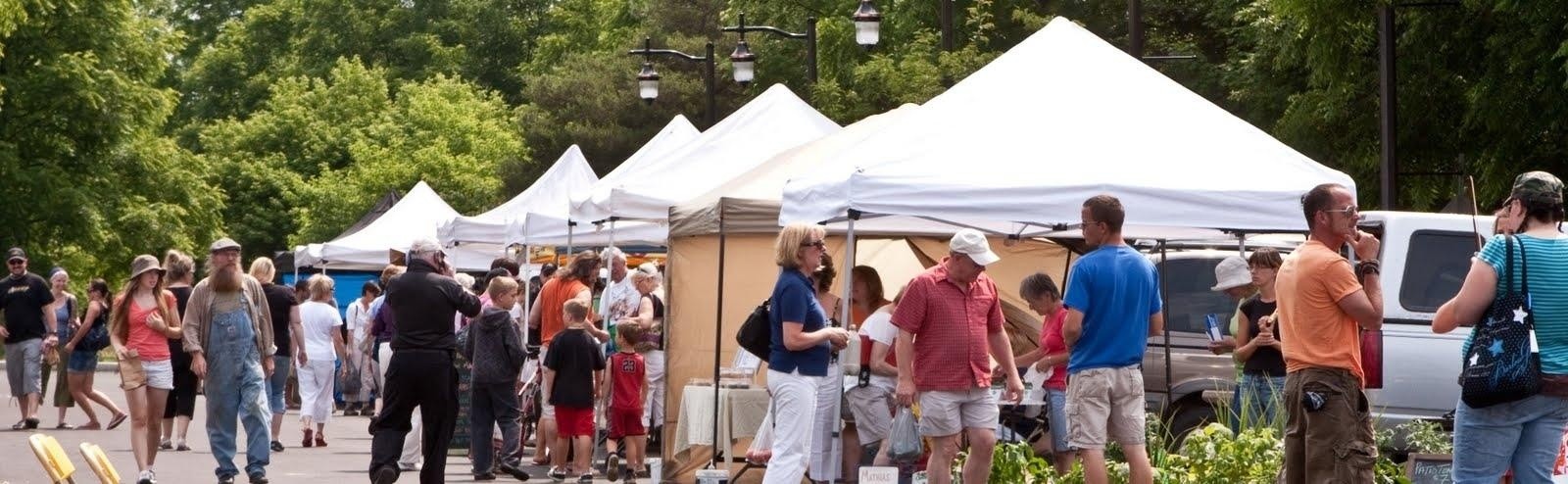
[314,431,328,447]
[302,427,313,448]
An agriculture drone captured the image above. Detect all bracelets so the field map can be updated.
[1358,259,1380,282]
[49,332,58,336]
[1048,356,1051,365]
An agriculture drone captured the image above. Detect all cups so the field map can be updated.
[799,239,825,249]
[9,259,24,265]
[1323,203,1360,218]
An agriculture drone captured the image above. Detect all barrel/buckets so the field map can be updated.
[55,422,74,431]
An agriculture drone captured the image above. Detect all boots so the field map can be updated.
[1333,209,1508,443]
[1109,252,1298,452]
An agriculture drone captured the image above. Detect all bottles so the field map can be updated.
[1209,253,1253,295]
[129,252,168,280]
[5,247,27,262]
[49,267,69,280]
[409,233,450,257]
[1501,169,1566,211]
[208,235,243,254]
[948,226,1002,266]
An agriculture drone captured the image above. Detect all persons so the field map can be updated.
[2,247,58,429]
[181,238,277,483]
[39,268,81,429]
[761,219,850,484]
[809,253,843,484]
[1207,256,1260,439]
[109,254,182,484]
[1061,194,1164,483]
[890,228,1026,484]
[362,264,421,472]
[1003,322,1049,441]
[369,238,481,483]
[161,251,199,450]
[343,280,381,416]
[1492,207,1568,484]
[64,278,128,430]
[992,273,1075,475]
[1235,247,1287,430]
[844,286,905,466]
[1274,183,1383,484]
[844,265,898,484]
[456,248,666,482]
[1432,171,1568,484]
[289,274,346,447]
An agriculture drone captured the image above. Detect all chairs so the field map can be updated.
[160,436,173,449]
[500,462,529,482]
[176,439,192,451]
[137,469,158,483]
[474,472,497,480]
[248,473,268,483]
[75,421,102,430]
[547,464,566,483]
[578,471,593,484]
[604,450,619,483]
[269,439,284,452]
[106,410,128,430]
[11,415,40,430]
[623,468,638,483]
[343,407,375,417]
[216,477,234,484]
[372,467,394,483]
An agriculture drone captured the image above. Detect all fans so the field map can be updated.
[440,350,474,449]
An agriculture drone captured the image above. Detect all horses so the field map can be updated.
[439,260,445,267]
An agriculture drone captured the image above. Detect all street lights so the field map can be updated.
[335,352,361,397]
[520,380,543,426]
[735,290,772,364]
[1458,288,1546,410]
[70,310,111,350]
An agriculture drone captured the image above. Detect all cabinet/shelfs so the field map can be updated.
[842,323,862,378]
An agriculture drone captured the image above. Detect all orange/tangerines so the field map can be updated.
[465,348,542,474]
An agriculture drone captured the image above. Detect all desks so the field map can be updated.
[626,35,715,132]
[845,0,954,93]
[722,7,819,97]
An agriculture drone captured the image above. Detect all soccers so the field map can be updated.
[746,449,772,463]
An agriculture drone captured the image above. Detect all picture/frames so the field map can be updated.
[671,384,817,484]
[956,401,1050,453]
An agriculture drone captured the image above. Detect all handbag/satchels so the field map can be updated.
[1358,259,1380,282]
